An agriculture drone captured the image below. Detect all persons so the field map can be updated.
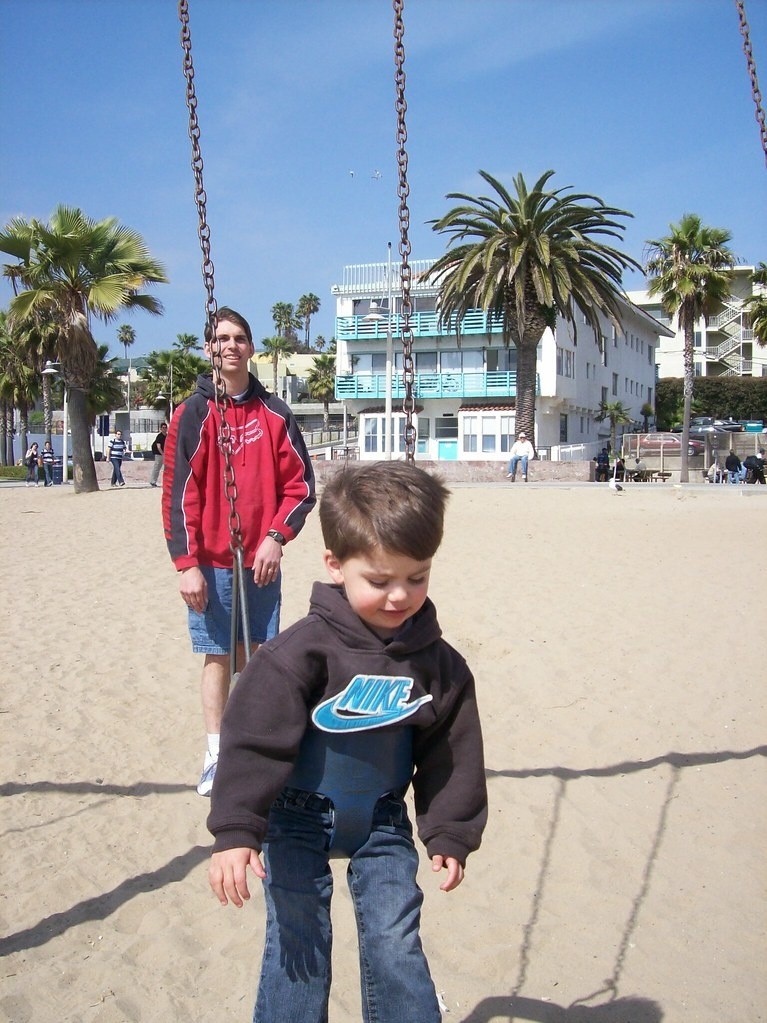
[726,449,742,484]
[615,458,625,479]
[160,304,316,797]
[106,430,127,488]
[506,430,534,482]
[594,447,610,483]
[25,441,55,487]
[707,447,766,484]
[633,458,646,482]
[150,423,168,487]
[206,461,490,1023]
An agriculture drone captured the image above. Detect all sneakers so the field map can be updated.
[197,750,219,795]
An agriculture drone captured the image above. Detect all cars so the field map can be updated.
[678,425,733,450]
[622,432,701,457]
[695,417,765,433]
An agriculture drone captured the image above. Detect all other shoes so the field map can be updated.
[522,475,526,479]
[506,473,514,477]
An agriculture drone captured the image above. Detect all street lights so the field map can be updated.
[40,360,70,485]
[155,365,174,424]
[362,239,393,463]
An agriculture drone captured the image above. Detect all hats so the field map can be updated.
[519,433,525,438]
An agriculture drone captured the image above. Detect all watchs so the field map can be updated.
[267,531,286,546]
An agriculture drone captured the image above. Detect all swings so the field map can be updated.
[171,0,419,858]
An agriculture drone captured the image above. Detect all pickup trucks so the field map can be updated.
[670,420,742,435]
